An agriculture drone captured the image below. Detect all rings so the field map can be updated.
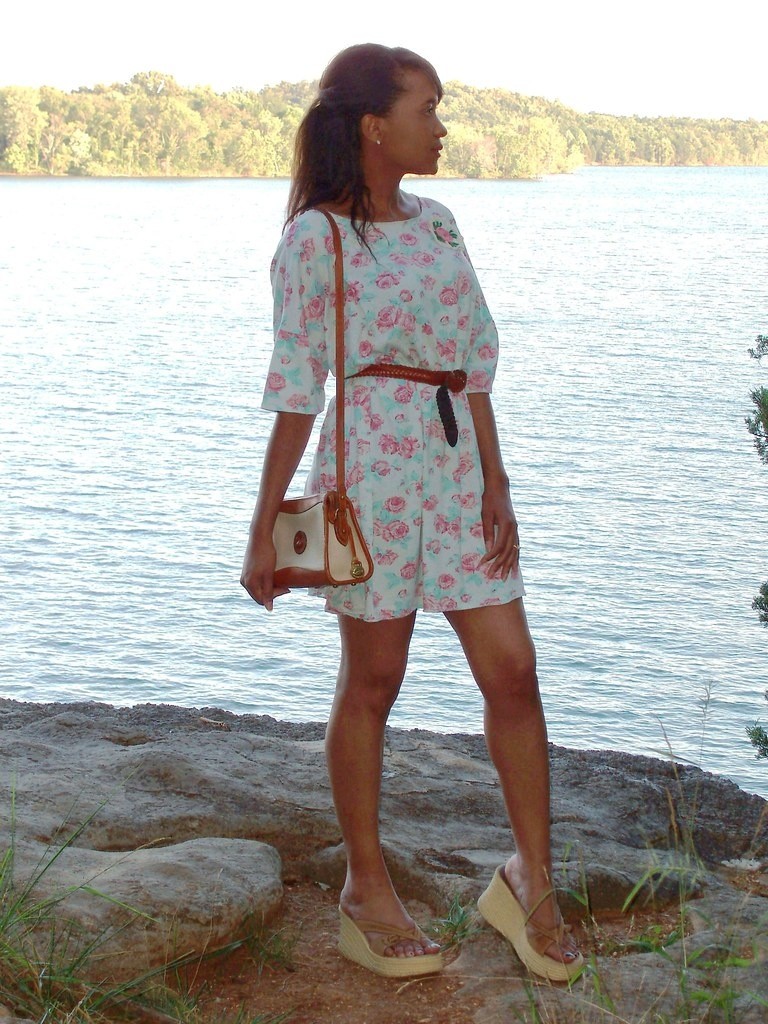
[513,545,517,549]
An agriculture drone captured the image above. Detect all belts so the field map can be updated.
[340,362,468,447]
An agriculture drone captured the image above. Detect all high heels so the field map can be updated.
[477,861,586,983]
[334,901,446,979]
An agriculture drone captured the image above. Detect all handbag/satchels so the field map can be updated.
[254,490,375,588]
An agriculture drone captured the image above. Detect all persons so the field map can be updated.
[240,43,582,981]
